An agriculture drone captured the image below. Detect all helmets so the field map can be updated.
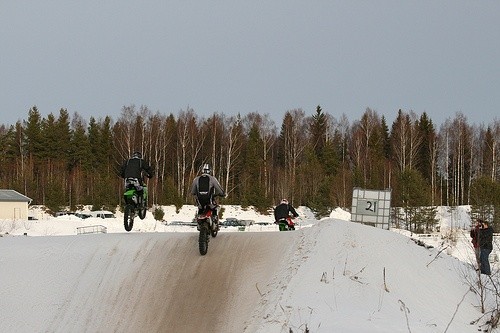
[131,151,142,159]
[201,164,210,173]
[280,199,288,205]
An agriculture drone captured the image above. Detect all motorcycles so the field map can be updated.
[274,215,295,231]
[197,194,226,255]
[124,172,155,231]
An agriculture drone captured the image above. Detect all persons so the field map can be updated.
[120,151,153,210]
[274,199,298,230]
[191,162,224,231]
[478,220,494,275]
[470,219,485,271]
